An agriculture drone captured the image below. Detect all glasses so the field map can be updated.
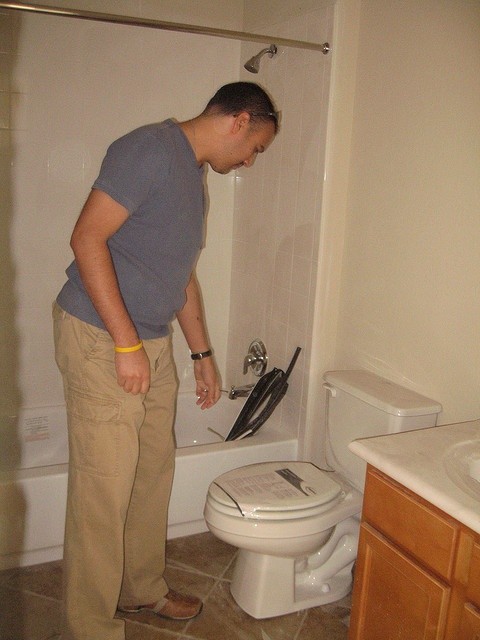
[232,110,283,128]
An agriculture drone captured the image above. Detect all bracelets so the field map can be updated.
[191,348,212,360]
[113,340,143,353]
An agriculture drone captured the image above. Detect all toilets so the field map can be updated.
[203,368,443,620]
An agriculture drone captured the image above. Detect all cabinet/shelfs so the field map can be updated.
[345,461,479,640]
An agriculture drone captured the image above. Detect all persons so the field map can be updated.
[52,82,279,639]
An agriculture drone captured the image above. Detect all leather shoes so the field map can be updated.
[118,588,202,620]
